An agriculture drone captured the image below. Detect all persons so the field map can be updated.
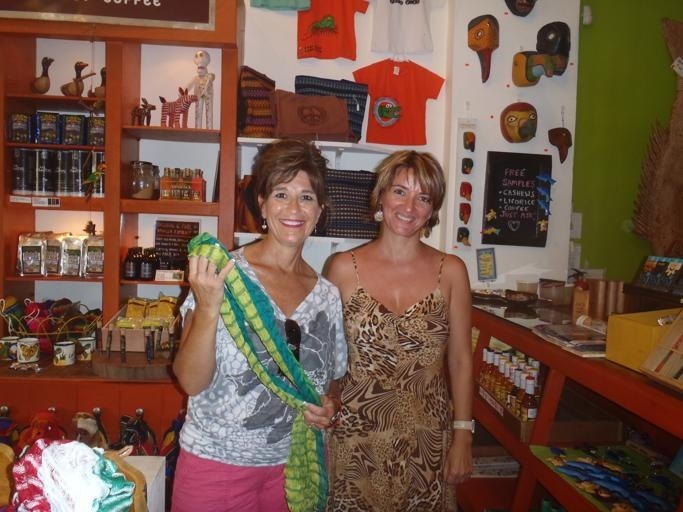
[321,149,476,512]
[171,139,348,512]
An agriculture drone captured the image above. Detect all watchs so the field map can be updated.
[453,419,476,434]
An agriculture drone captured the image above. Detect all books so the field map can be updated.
[471,446,520,478]
[531,324,607,358]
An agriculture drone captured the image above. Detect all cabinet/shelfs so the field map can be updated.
[0,1,243,457]
[443,278,682,511]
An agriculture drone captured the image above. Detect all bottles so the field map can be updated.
[479,347,541,422]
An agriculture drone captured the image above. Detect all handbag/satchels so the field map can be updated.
[295,75,368,144]
[276,89,350,142]
[240,64,276,139]
[236,173,266,234]
[316,169,379,240]
